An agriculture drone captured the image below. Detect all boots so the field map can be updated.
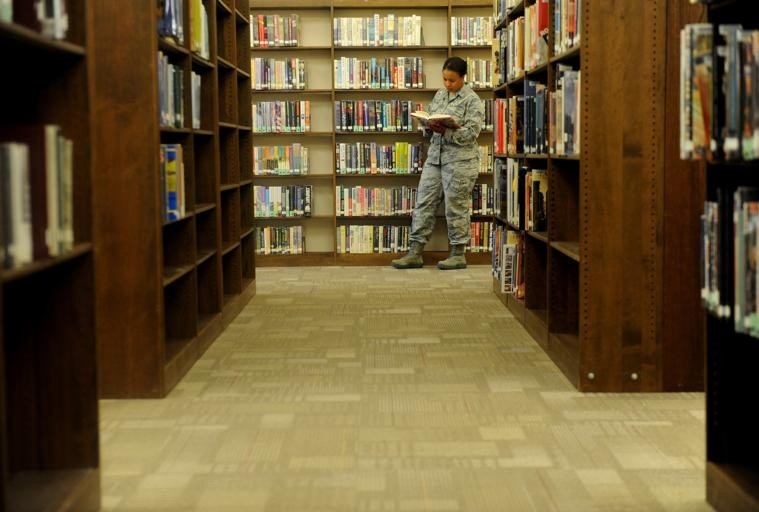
[437,245,468,269]
[391,241,425,269]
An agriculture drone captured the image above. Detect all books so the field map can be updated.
[678,23,758,340]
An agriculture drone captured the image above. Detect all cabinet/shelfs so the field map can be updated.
[92,2,250,398]
[0,0,99,510]
[492,2,666,392]
[667,2,704,392]
[705,2,759,508]
[249,1,495,263]
